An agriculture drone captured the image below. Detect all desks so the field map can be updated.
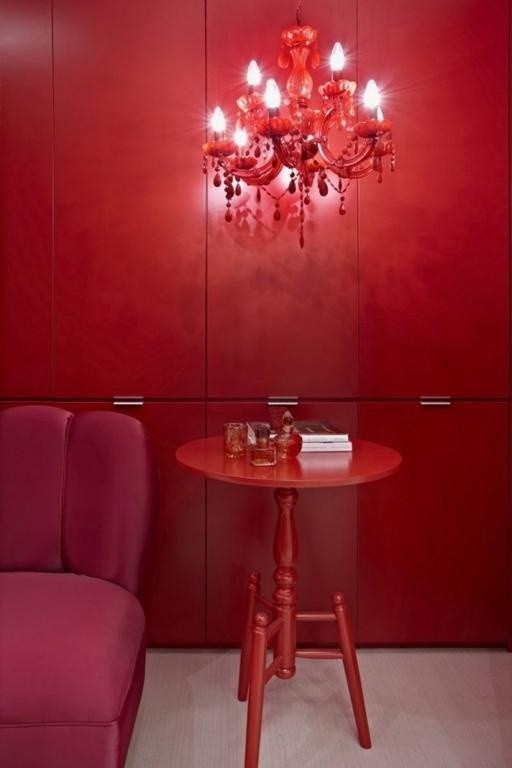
[175,436,401,768]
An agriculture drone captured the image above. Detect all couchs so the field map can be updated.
[0,405,158,768]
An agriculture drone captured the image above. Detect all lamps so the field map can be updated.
[200,0,397,249]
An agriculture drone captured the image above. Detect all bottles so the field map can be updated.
[273,415,303,460]
[247,426,276,467]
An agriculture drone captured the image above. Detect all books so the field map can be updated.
[245,420,349,442]
[247,432,352,454]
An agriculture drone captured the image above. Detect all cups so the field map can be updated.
[223,422,247,458]
